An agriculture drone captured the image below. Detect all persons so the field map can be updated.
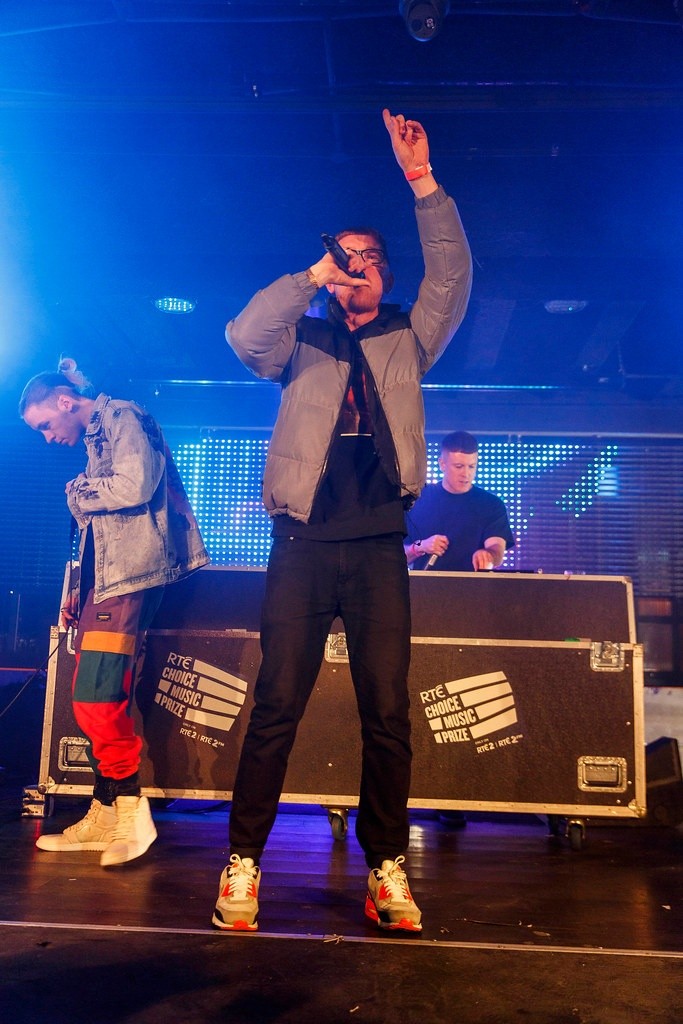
[18,354,211,866]
[403,430,515,572]
[212,109,475,933]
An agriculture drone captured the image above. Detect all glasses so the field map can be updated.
[352,248,389,264]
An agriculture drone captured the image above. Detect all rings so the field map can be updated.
[60,607,69,615]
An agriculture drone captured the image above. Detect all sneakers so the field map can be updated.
[365,855,422,932]
[212,854,261,931]
[36,798,118,852]
[100,796,157,866]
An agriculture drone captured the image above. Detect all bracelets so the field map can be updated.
[305,268,319,289]
[410,539,426,557]
[404,162,432,181]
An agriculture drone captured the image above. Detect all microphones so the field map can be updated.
[320,234,365,279]
[71,515,78,532]
[424,554,438,570]
